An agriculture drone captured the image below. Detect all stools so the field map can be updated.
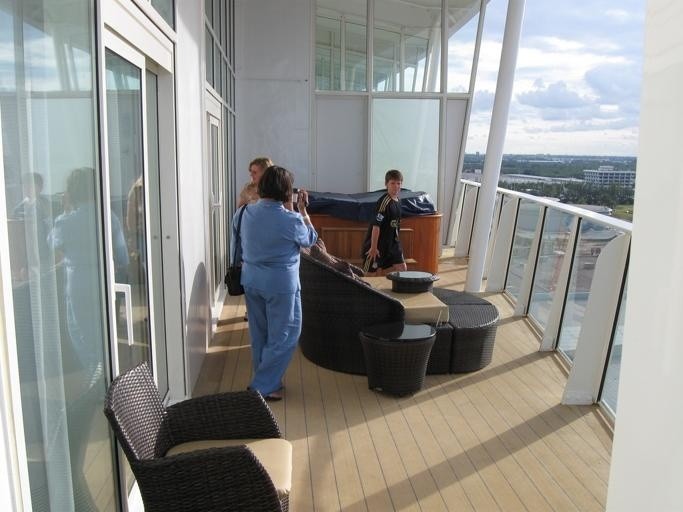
[358,323,438,398]
[433,286,499,373]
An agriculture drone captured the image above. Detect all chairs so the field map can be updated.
[102,361,295,512]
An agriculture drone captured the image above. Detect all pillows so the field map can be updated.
[310,237,373,288]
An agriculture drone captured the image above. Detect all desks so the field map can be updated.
[310,212,445,278]
[386,270,441,295]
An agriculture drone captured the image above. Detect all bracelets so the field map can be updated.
[302,214,310,220]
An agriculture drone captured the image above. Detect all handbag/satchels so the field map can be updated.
[224,264,246,295]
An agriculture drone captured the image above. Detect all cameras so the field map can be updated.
[293,188,300,203]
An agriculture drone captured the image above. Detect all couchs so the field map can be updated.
[299,251,452,375]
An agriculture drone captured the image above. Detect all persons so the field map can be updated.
[237,155,276,324]
[48,168,126,385]
[228,165,318,403]
[361,169,408,277]
[10,171,55,261]
[126,174,144,281]
